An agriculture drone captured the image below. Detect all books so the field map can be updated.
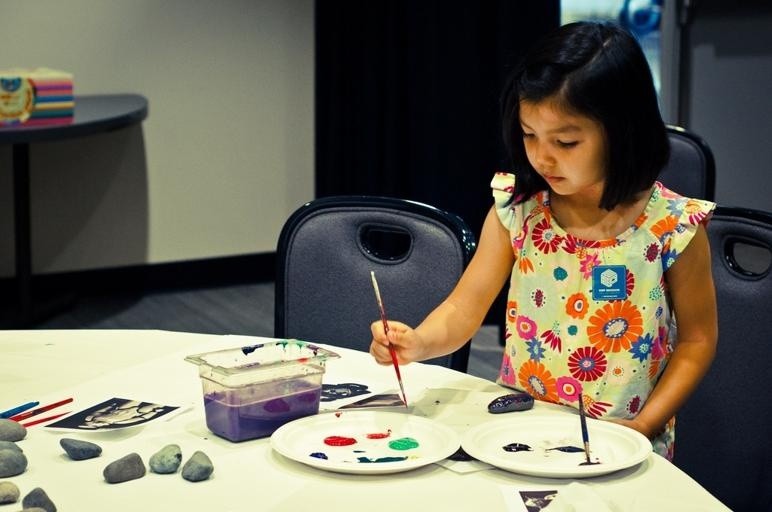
[0,70,75,127]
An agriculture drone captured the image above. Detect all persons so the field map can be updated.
[369,21,720,461]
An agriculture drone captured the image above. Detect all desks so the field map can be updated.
[2,92,146,324]
[0,329,735,511]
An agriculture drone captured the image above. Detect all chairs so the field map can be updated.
[272,197,479,379]
[659,124,716,205]
[669,206,771,511]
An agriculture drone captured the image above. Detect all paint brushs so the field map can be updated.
[370,271,407,406]
[0,398,73,428]
[578,393,592,463]
